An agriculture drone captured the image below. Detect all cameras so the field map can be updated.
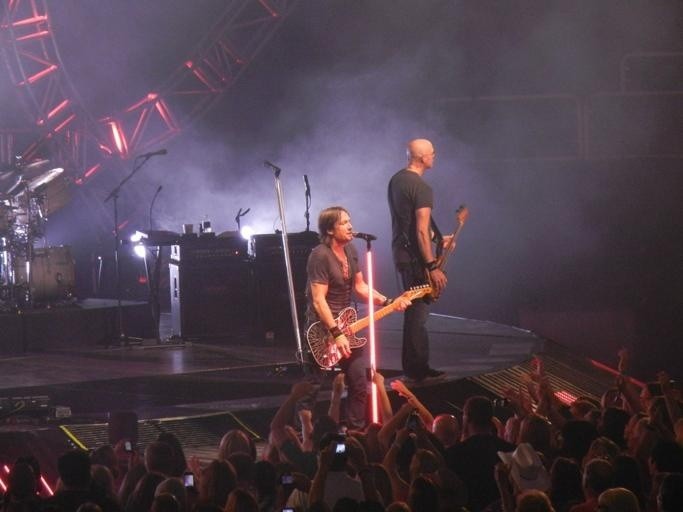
[282,508,294,512]
[336,443,350,456]
[281,475,296,484]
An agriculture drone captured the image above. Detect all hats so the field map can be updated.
[494,440,553,498]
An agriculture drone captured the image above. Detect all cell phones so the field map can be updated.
[124,441,132,454]
[184,472,193,489]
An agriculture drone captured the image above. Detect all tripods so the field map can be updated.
[95,155,151,345]
[0,191,53,314]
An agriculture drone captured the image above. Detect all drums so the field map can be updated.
[13,246,76,300]
[13,166,72,217]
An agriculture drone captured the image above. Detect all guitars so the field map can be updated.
[425,205,469,303]
[304,284,433,368]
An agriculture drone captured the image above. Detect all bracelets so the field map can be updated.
[330,327,344,340]
[384,299,391,305]
[426,261,436,270]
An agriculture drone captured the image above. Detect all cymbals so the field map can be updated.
[0,159,49,179]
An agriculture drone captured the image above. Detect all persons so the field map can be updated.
[0,348,682,512]
[387,138,455,379]
[298,207,412,432]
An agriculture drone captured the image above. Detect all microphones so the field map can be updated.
[303,175,310,197]
[139,149,167,154]
[263,162,280,172]
[352,232,377,240]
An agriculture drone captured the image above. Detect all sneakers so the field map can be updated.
[399,366,450,385]
